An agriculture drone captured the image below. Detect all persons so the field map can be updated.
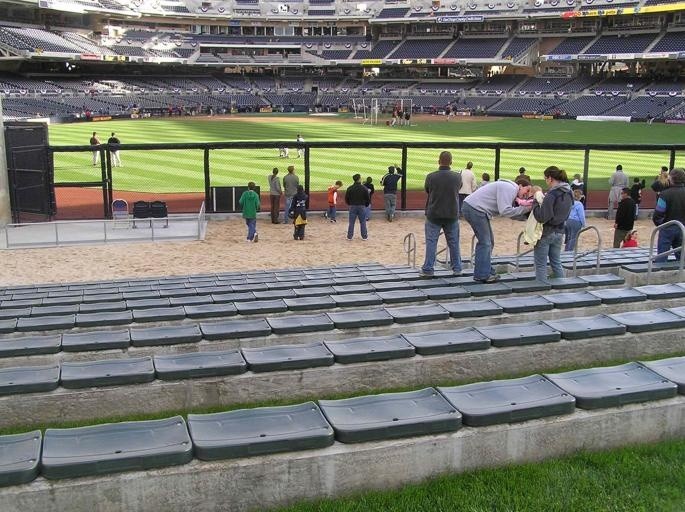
[108,132,123,168]
[570,173,586,203]
[628,178,646,219]
[279,147,288,158]
[159,101,222,117]
[418,150,464,276]
[323,181,343,222]
[233,102,330,113]
[380,163,404,222]
[652,168,685,270]
[37,101,152,119]
[531,166,575,281]
[654,166,668,181]
[296,133,306,158]
[237,183,262,242]
[477,172,491,192]
[345,173,369,241]
[516,185,544,245]
[362,177,375,221]
[622,229,638,247]
[283,166,300,225]
[287,184,309,240]
[268,168,283,225]
[352,100,437,126]
[515,167,532,221]
[608,164,629,218]
[650,172,670,205]
[613,187,637,248]
[444,95,684,128]
[458,162,478,219]
[90,132,100,164]
[564,190,587,252]
[461,178,531,283]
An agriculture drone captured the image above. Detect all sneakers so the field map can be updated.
[474,274,500,282]
[453,270,462,276]
[420,270,433,276]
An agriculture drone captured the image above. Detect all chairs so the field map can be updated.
[111,199,169,228]
